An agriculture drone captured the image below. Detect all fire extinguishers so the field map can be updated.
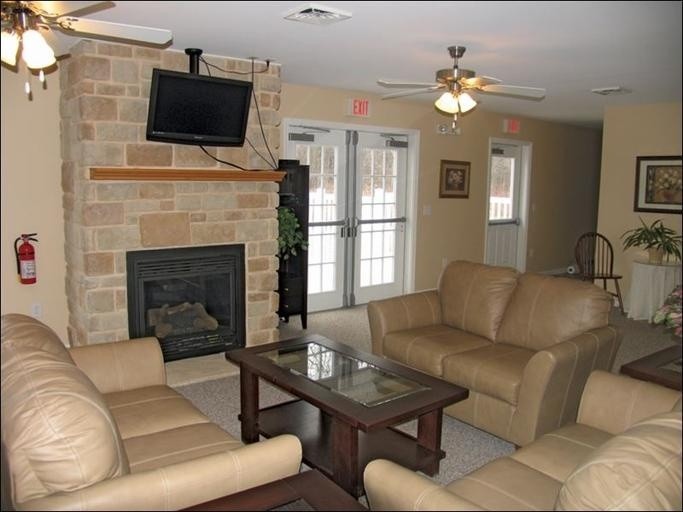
[14,233,38,284]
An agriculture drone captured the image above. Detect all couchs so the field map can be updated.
[1,314,303,511]
[363,369,683,511]
[367,261,625,451]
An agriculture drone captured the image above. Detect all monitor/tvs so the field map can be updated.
[145,68,254,148]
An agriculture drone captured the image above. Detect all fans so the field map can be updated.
[377,46,546,100]
[1,1,173,45]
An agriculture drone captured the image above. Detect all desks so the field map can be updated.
[622,345,682,391]
[179,468,369,511]
[626,260,682,324]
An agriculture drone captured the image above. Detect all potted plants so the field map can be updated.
[279,206,309,329]
[620,216,682,265]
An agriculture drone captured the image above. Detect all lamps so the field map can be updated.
[435,82,477,129]
[1,7,71,94]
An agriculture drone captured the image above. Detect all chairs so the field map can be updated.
[574,232,624,315]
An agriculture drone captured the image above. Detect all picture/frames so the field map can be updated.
[634,156,683,214]
[439,160,471,199]
